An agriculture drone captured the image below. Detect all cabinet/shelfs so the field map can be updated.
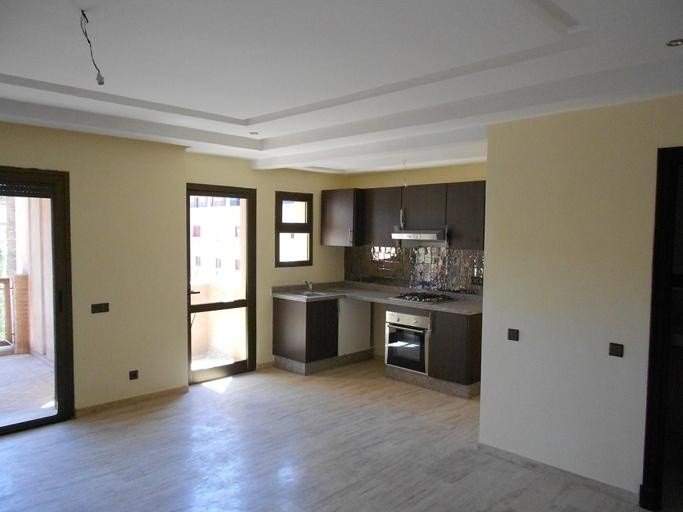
[446,180,486,252]
[320,188,357,247]
[355,188,400,247]
[400,183,447,230]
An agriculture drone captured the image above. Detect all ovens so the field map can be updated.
[383,310,431,377]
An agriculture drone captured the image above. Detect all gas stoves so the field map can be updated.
[387,291,457,304]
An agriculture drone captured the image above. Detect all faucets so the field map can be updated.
[304,281,313,292]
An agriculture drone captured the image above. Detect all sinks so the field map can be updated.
[290,293,324,298]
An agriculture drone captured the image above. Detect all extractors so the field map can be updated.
[391,230,444,242]
